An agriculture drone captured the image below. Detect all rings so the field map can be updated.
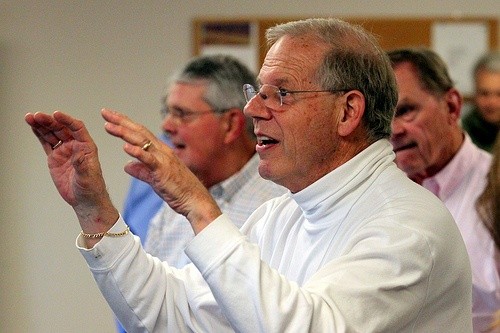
[52,140,61,149]
[142,141,153,151]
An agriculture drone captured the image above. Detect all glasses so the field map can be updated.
[243,84,352,109]
[160,105,231,126]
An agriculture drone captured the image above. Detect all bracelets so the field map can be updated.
[81,226,130,239]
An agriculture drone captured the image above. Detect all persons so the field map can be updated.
[144,54,291,267]
[23,18,475,333]
[381,48,500,333]
